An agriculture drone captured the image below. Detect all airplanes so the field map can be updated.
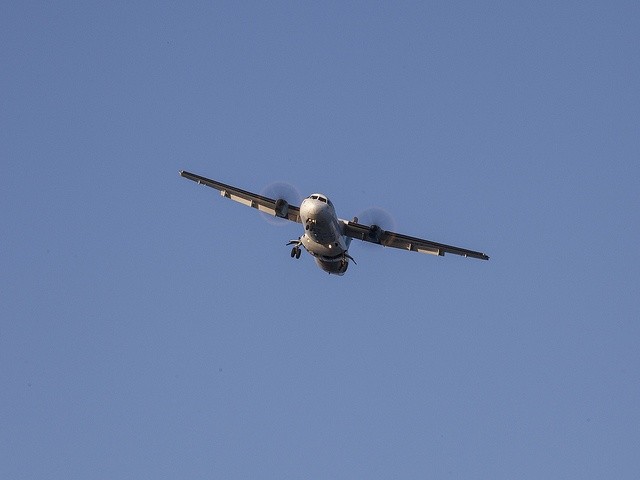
[179,170,489,275]
